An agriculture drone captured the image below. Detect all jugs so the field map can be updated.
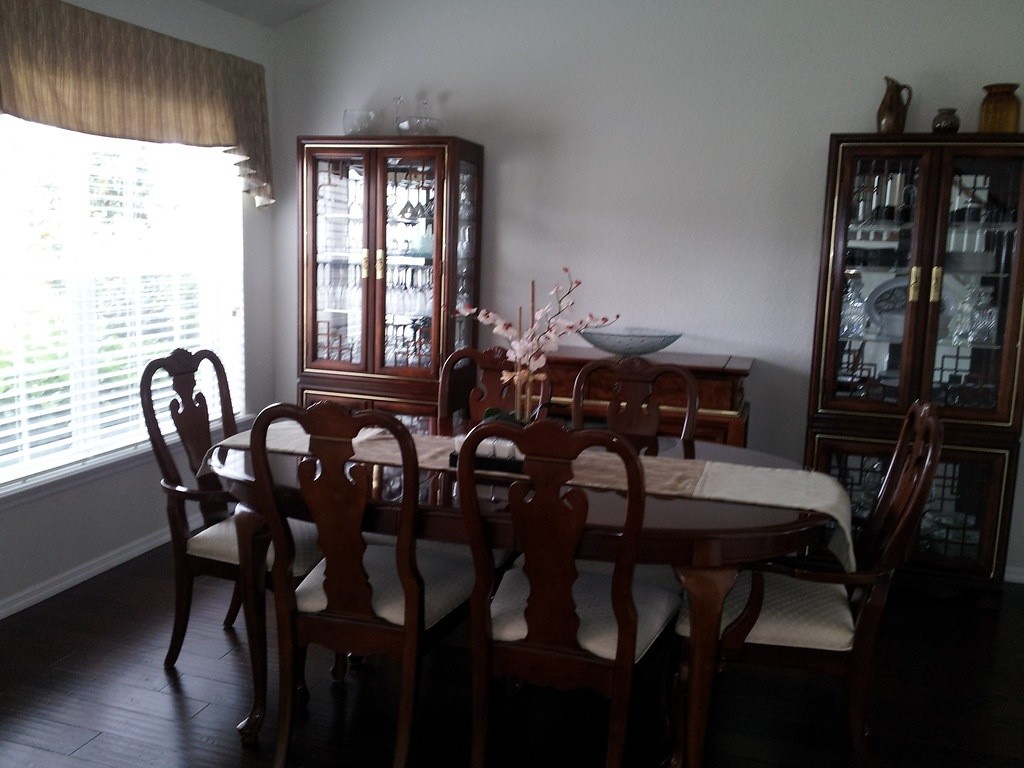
[876,76,913,134]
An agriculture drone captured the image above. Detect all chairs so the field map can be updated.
[141,347,945,768]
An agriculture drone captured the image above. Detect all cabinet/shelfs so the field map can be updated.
[294,135,486,436]
[801,132,1024,583]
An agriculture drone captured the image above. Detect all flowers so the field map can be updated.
[450,267,621,387]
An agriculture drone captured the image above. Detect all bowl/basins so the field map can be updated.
[397,117,444,135]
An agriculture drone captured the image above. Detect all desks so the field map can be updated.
[207,412,844,768]
[508,346,754,448]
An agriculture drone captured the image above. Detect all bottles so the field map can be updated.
[932,107,960,134]
[979,82,1021,132]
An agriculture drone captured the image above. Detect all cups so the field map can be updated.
[455,434,526,461]
[343,102,383,135]
